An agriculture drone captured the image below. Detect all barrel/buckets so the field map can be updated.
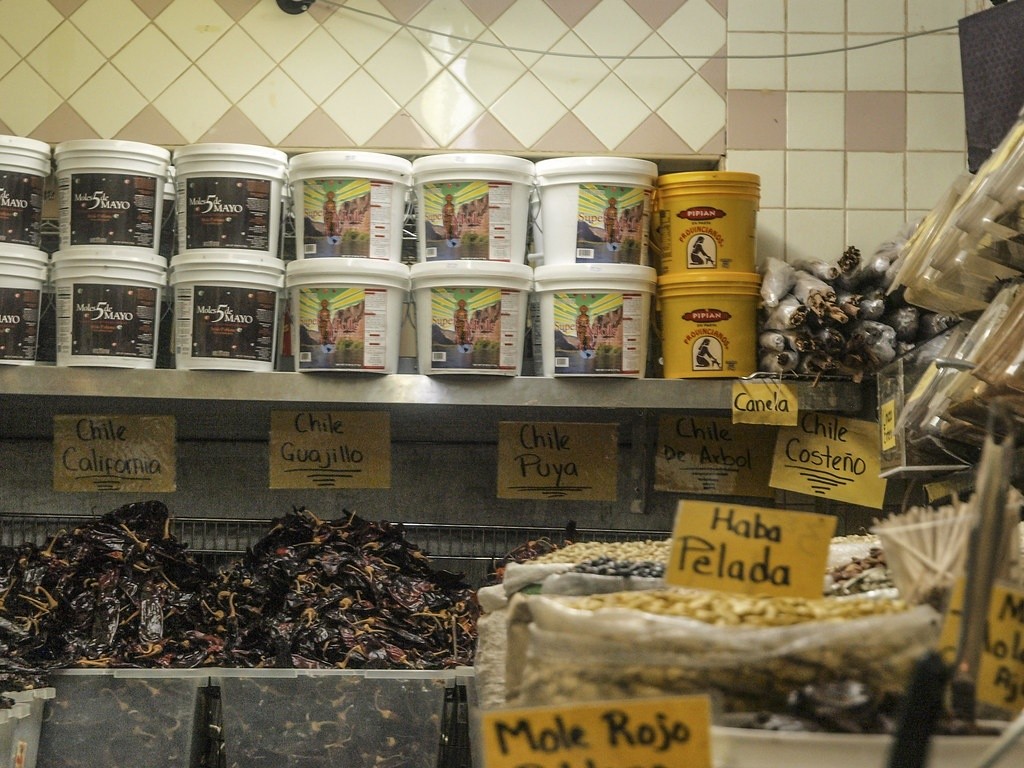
[532,156,658,265]
[50,248,167,369]
[412,153,536,265]
[168,247,286,371]
[529,261,656,379]
[409,257,533,377]
[655,169,761,278]
[172,144,289,259]
[286,257,410,377]
[286,150,413,265]
[52,138,171,255]
[0,240,49,367]
[0,135,52,251]
[656,272,764,380]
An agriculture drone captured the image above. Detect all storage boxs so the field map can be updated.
[37,668,209,768]
[1,687,56,768]
[209,666,456,768]
[0,703,31,768]
